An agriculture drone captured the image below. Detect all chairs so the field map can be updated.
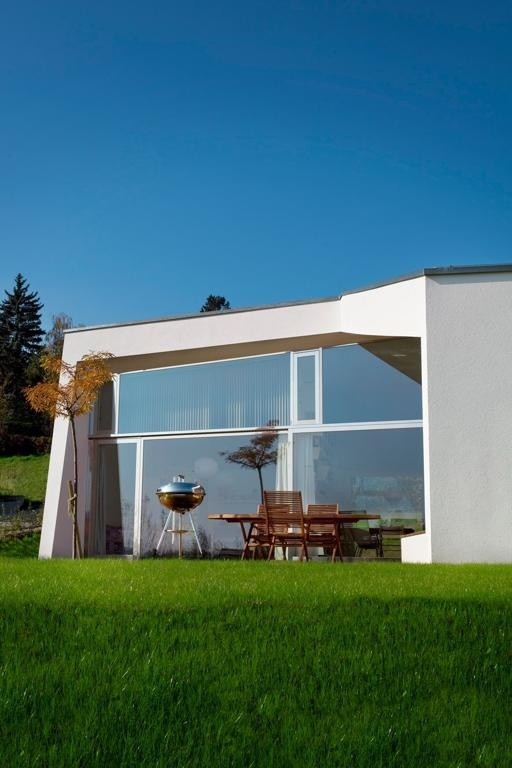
[340,510,415,558]
[208,491,381,563]
